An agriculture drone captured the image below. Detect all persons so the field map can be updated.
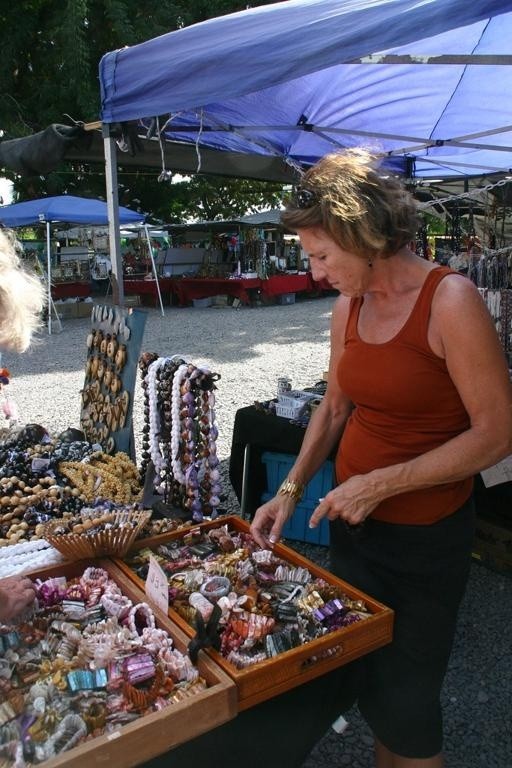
[249,144,511,768]
[1,224,49,356]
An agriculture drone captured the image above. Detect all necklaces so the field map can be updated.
[128,351,222,522]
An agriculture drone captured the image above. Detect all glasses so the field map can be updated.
[290,185,323,209]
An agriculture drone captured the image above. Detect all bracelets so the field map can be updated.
[276,478,307,503]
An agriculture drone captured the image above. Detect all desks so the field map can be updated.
[231,379,328,521]
[46,270,341,307]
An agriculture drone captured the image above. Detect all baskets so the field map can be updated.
[276,389,323,421]
[40,510,154,559]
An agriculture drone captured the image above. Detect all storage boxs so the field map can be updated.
[50,302,79,320]
[79,302,94,318]
[259,492,333,547]
[262,452,336,500]
